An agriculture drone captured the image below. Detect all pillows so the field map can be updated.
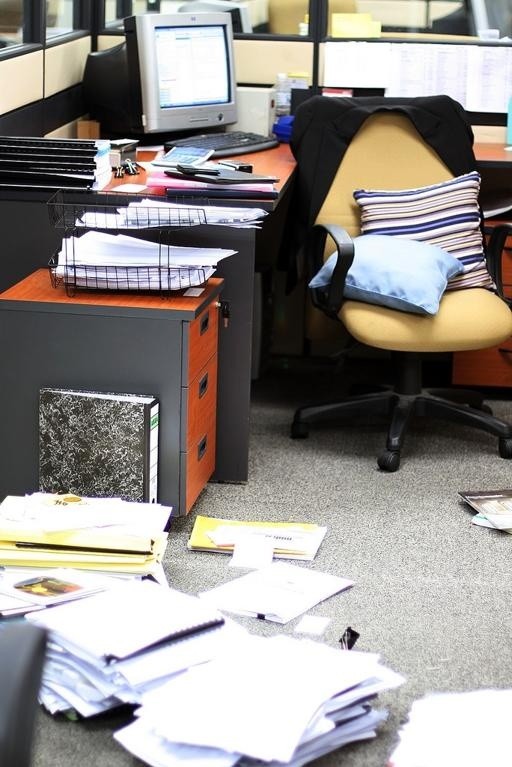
[309,234,465,315]
[353,171,496,290]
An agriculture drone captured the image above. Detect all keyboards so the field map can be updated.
[164,130,279,160]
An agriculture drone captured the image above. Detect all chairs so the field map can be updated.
[291,95,512,471]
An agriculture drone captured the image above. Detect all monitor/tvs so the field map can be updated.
[79,10,238,147]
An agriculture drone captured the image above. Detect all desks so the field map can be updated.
[295,141,512,396]
[1,128,306,485]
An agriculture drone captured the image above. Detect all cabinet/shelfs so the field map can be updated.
[3,266,225,537]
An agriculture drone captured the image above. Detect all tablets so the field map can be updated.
[150,145,215,169]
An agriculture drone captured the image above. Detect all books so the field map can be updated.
[112,631,407,767]
[0,567,125,616]
[199,562,355,626]
[0,491,173,588]
[146,164,281,199]
[457,489,512,535]
[0,136,113,191]
[186,515,327,561]
[109,138,140,168]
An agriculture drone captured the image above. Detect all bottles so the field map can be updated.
[274,73,291,120]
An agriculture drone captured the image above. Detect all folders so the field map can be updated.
[38,388,159,505]
[0,138,111,187]
